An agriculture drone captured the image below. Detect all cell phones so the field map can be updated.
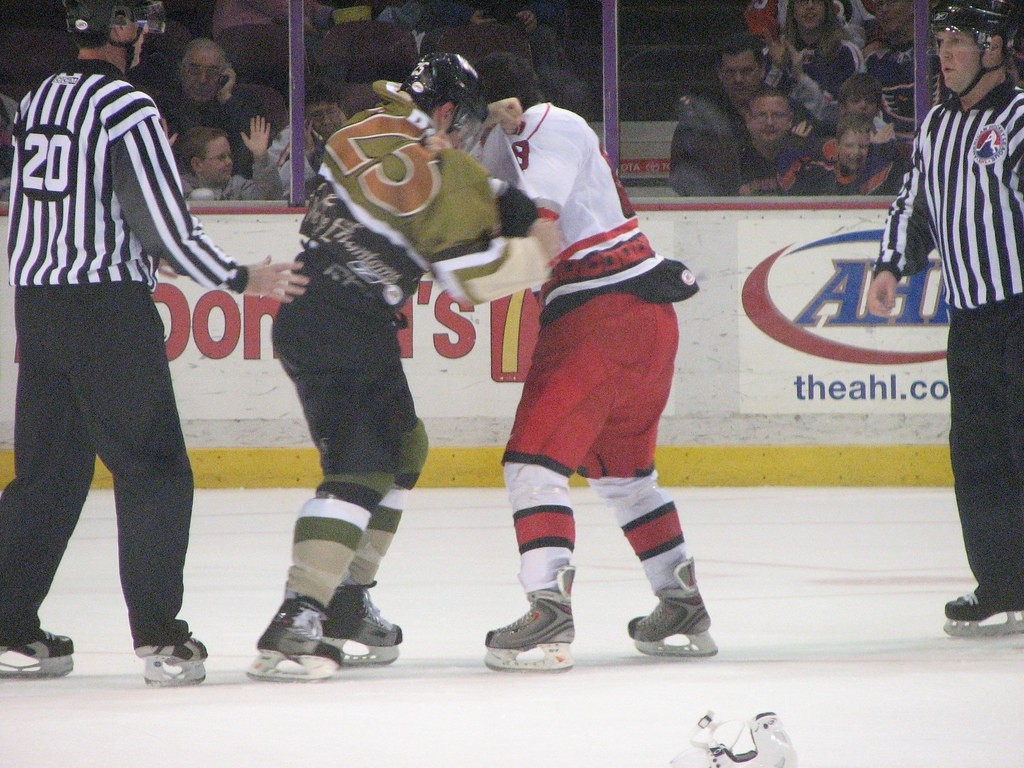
[219,75,229,85]
[306,125,324,146]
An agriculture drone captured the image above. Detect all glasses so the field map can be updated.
[182,66,220,79]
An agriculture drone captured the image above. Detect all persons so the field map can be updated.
[868,0,1024,622]
[154,39,277,180]
[161,115,283,201]
[719,87,837,196]
[744,0,896,141]
[0,0,311,662]
[423,52,711,649]
[255,52,564,667]
[450,0,560,68]
[860,0,948,145]
[792,114,904,194]
[266,76,347,198]
[669,33,828,198]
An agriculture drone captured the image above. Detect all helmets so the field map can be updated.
[59,0,167,46]
[929,1,1022,50]
[399,52,490,124]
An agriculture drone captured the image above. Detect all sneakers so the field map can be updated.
[0,628,76,680]
[241,597,346,681]
[133,633,211,689]
[627,591,718,659]
[482,589,577,673]
[944,588,1024,638]
[321,581,404,667]
